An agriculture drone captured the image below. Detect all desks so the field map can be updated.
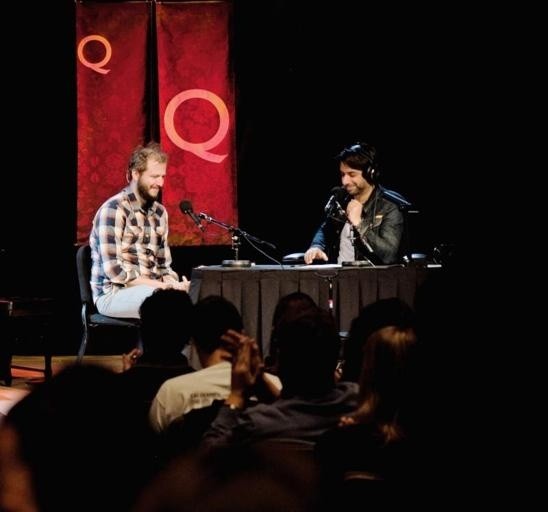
[189,263,422,360]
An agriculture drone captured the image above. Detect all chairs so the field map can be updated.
[75,243,146,366]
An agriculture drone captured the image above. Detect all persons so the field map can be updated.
[87,140,192,320]
[302,143,406,268]
[1,288,547,510]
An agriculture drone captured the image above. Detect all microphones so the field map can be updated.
[180,201,205,231]
[324,186,343,214]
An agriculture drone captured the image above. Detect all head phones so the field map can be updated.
[354,145,380,182]
